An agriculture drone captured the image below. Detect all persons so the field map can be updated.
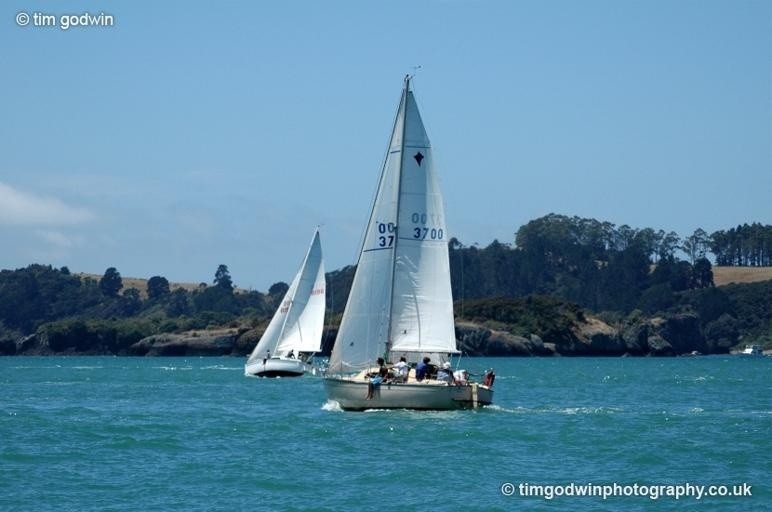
[415,357,432,382]
[435,362,454,384]
[385,355,411,382]
[364,356,388,402]
[262,349,271,365]
[287,349,295,359]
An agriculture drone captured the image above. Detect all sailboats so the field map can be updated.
[324,69,506,414]
[237,223,340,377]
